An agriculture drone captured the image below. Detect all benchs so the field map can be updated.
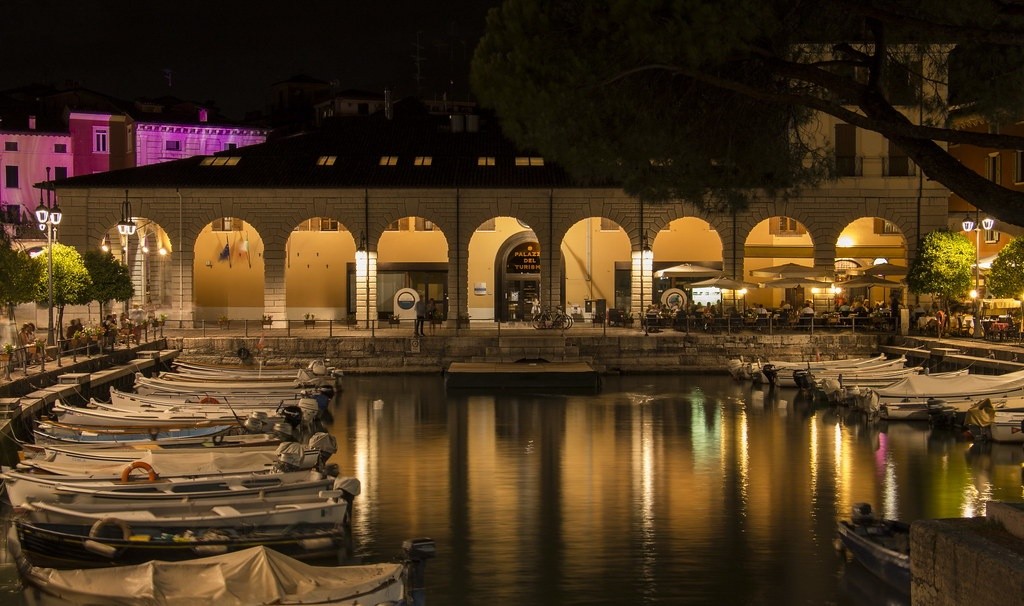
[212,506,241,516]
[164,490,175,493]
[132,445,163,450]
[206,416,220,419]
[81,431,99,436]
[229,485,248,490]
[168,478,187,482]
[159,416,171,419]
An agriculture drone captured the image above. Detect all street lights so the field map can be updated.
[35,167,63,359]
[118,189,136,344]
[961,208,994,339]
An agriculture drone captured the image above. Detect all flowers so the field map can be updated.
[459,312,471,320]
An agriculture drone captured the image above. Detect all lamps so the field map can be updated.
[205,261,212,268]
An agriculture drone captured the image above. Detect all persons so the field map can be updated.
[532,296,540,319]
[426,298,437,337]
[16,304,146,363]
[641,293,1020,342]
[416,295,425,337]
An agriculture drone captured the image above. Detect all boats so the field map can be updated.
[727,353,1024,419]
[927,395,1024,433]
[964,398,1024,443]
[837,503,910,598]
[0,358,361,567]
[7,534,436,606]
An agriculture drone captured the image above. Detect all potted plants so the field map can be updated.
[261,315,273,325]
[0,314,169,361]
[217,313,231,325]
[432,313,448,328]
[389,314,400,324]
[303,312,315,325]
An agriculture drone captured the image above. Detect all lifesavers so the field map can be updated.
[237,347,250,360]
[89,517,130,540]
[121,461,156,483]
[199,398,219,404]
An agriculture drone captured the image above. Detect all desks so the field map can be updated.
[918,317,937,333]
[992,323,1009,341]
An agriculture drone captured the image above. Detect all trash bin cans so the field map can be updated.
[591,299,606,323]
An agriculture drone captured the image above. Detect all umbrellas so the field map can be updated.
[654,259,991,313]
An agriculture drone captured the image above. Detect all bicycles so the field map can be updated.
[531,305,572,330]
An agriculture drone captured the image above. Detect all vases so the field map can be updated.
[460,320,470,329]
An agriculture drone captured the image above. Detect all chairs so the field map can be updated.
[638,305,1021,342]
